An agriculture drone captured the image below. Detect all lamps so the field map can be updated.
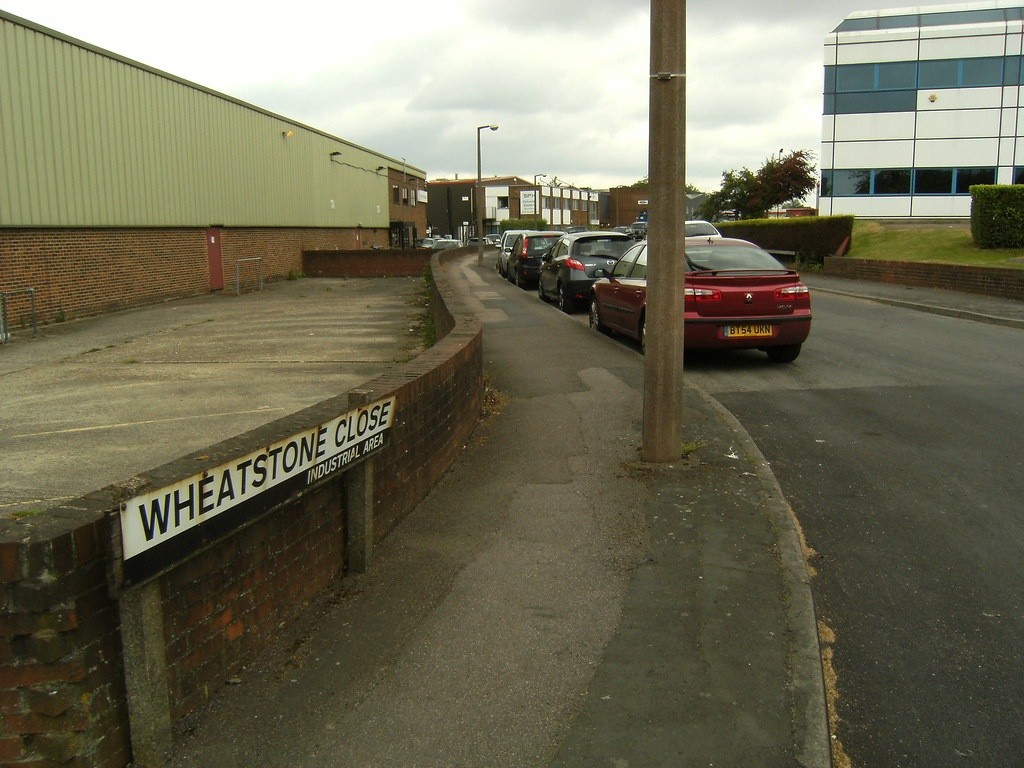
[329,151,341,156]
[377,165,386,171]
[283,129,293,138]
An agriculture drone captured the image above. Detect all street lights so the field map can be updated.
[534,173,547,214]
[477,123,500,266]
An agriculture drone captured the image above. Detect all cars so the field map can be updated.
[497,228,537,279]
[588,236,813,367]
[612,221,649,243]
[538,228,636,315]
[506,231,569,288]
[563,226,587,236]
[415,234,502,253]
[684,220,722,239]
